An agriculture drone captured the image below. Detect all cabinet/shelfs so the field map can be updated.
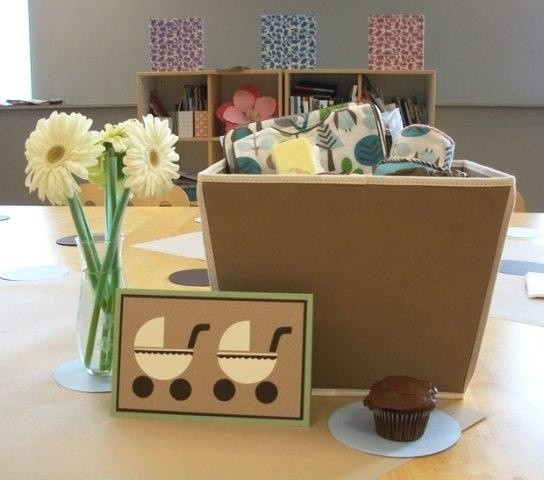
[135,68,283,201]
[284,70,437,153]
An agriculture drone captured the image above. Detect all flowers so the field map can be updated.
[26,110,183,377]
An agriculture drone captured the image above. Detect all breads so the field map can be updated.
[362,374,437,441]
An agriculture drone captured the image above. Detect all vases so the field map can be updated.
[56,235,129,395]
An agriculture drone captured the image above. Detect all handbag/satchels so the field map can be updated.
[222,100,389,176]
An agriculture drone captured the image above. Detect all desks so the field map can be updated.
[0,206,544,480]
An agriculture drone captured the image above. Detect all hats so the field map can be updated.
[372,122,457,176]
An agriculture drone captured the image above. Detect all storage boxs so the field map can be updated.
[195,158,518,400]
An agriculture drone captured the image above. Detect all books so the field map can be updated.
[148,81,208,117]
[288,83,429,127]
[7,98,63,107]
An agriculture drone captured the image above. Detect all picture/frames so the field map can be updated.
[111,286,314,429]
